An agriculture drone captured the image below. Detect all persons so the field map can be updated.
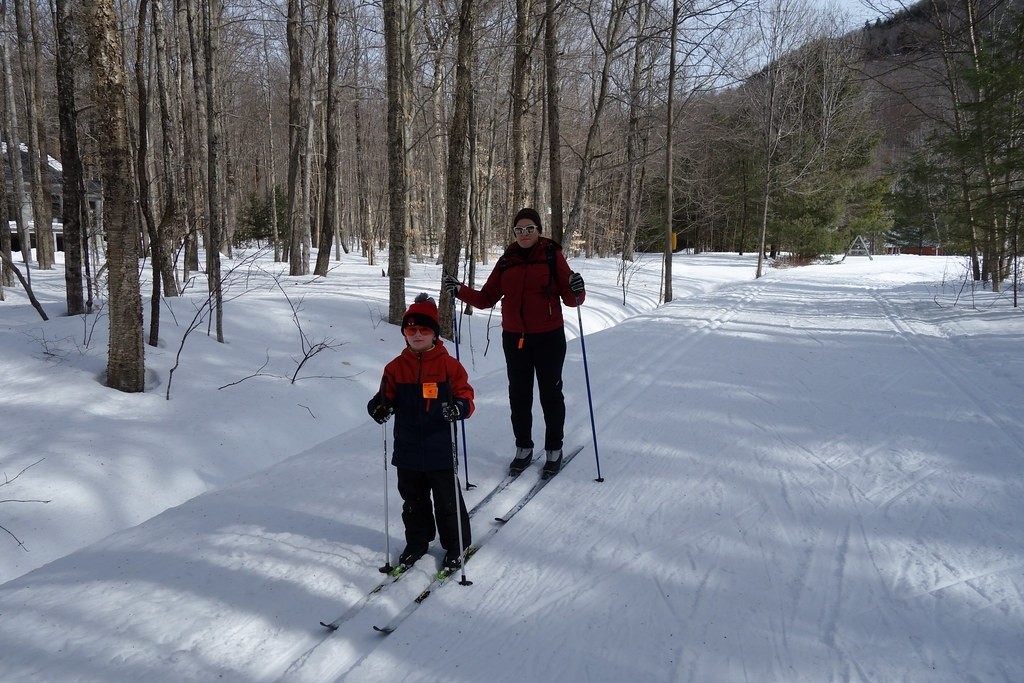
[368,292,475,567]
[442,207,586,474]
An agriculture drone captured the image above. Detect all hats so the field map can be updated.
[401,292,440,336]
[514,208,543,234]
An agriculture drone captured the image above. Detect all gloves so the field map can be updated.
[441,398,468,421]
[366,397,394,424]
[444,275,459,299]
[569,272,584,292]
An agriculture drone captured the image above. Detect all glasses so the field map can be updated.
[403,325,433,337]
[514,225,538,236]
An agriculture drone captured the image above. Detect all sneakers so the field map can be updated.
[510,447,533,471]
[399,542,428,565]
[543,448,564,475]
[443,547,469,571]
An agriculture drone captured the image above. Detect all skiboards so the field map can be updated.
[465,438,585,523]
[318,519,499,634]
